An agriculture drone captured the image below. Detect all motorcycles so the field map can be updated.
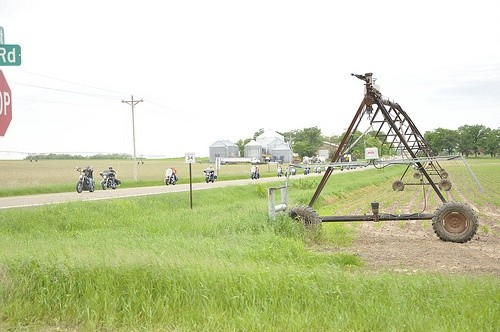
[277,165,357,177]
[100,169,122,190]
[75,168,96,193]
[165,168,178,185]
[204,167,217,183]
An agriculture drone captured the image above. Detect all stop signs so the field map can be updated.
[0,68,13,137]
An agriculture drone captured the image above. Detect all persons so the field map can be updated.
[210,166,214,170]
[255,166,260,176]
[171,167,177,181]
[279,166,283,173]
[77,164,94,192]
[101,167,116,189]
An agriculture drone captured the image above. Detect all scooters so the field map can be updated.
[250,166,259,179]
